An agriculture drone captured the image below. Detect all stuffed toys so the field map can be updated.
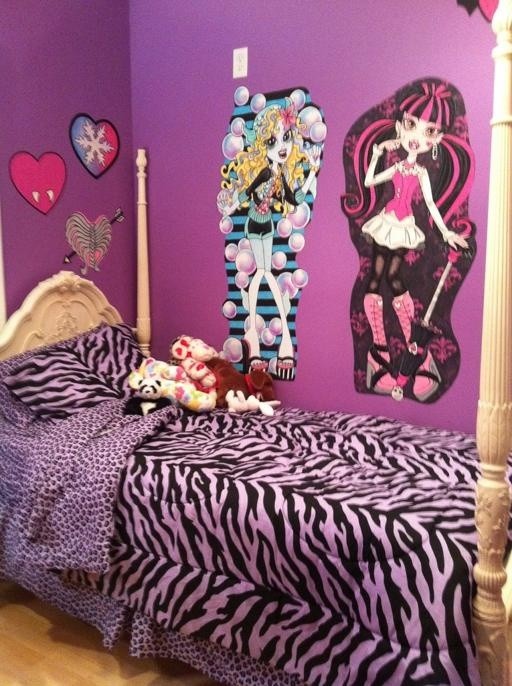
[124,334,281,416]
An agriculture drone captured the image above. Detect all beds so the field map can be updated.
[0,269,512,685]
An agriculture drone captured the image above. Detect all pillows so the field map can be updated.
[0,320,137,430]
